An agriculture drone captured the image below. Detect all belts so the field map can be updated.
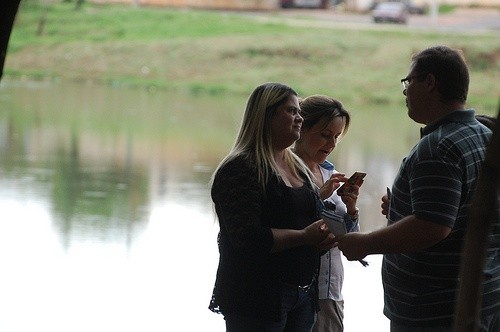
[286,280,316,292]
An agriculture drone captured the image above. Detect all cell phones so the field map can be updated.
[386,187,391,220]
[337,171,367,197]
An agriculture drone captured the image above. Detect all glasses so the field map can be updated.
[401,75,427,89]
[323,200,336,213]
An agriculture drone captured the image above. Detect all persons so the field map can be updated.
[338,46,500,332]
[208,82,334,332]
[287,96,364,332]
